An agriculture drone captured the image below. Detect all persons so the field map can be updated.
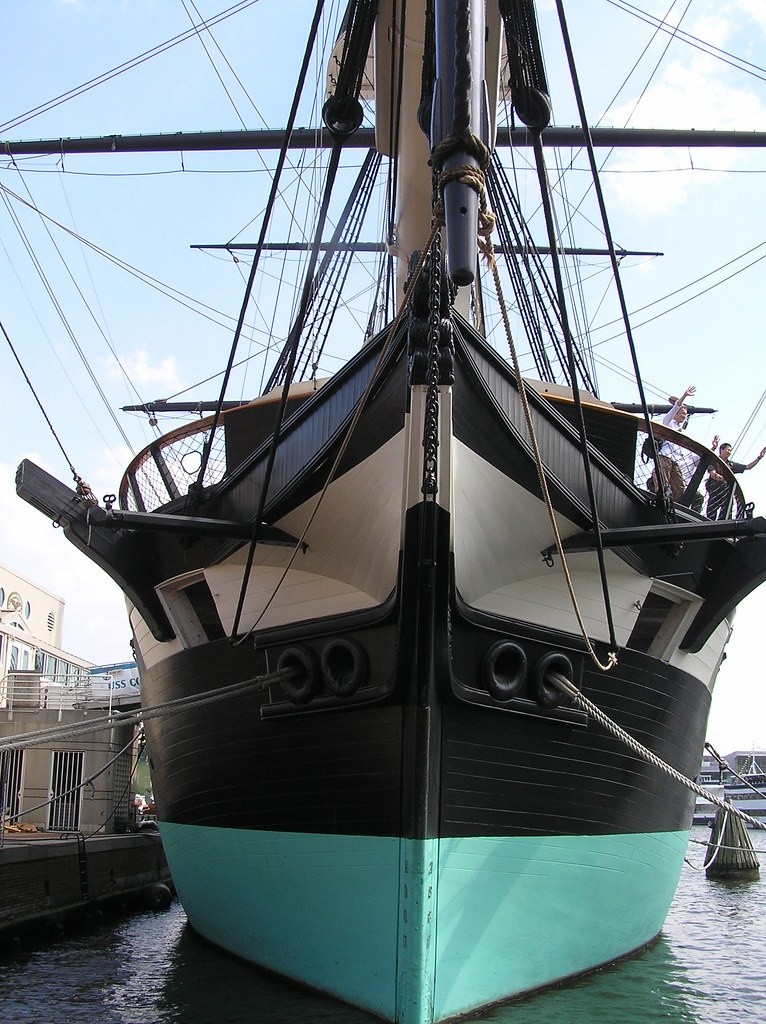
[654,385,697,501]
[706,443,766,521]
[682,434,720,511]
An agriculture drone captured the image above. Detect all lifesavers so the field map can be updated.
[484,639,573,709]
[142,882,173,912]
[277,637,365,702]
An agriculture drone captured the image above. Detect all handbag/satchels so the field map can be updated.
[642,437,662,464]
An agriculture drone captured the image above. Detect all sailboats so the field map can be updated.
[0,0,766,1024]
